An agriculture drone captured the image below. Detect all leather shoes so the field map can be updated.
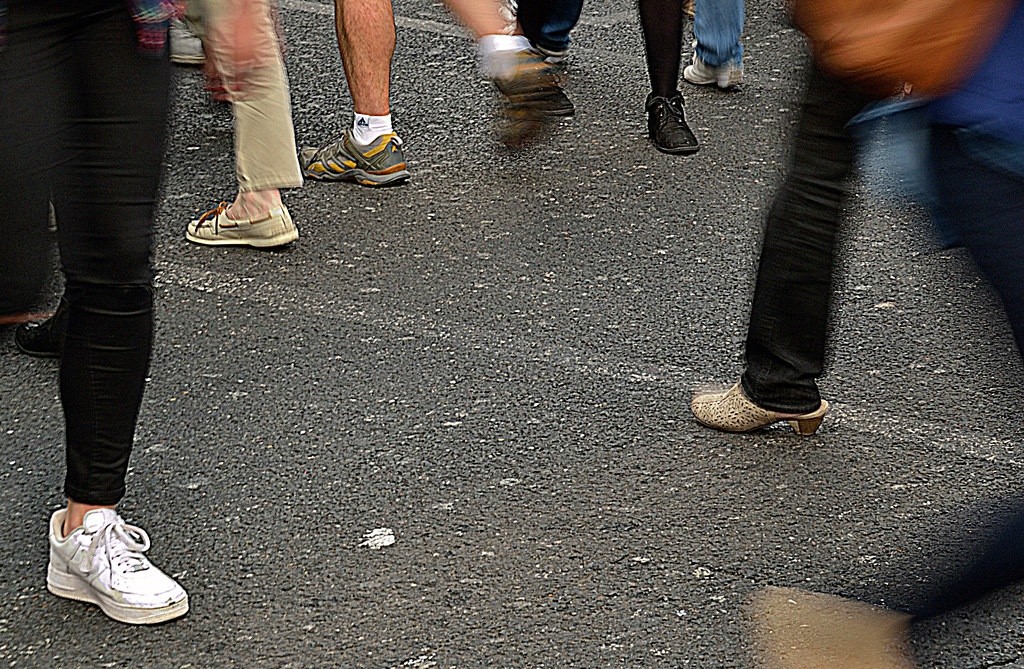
[15,305,69,359]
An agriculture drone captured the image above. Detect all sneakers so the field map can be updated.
[299,129,411,188]
[172,20,206,63]
[684,40,746,89]
[488,63,562,148]
[44,508,189,625]
[497,63,573,120]
[183,199,300,250]
[644,91,700,154]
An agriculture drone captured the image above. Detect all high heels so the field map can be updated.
[692,379,830,439]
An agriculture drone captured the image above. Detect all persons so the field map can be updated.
[0,0,746,627]
[689,0,1020,666]
